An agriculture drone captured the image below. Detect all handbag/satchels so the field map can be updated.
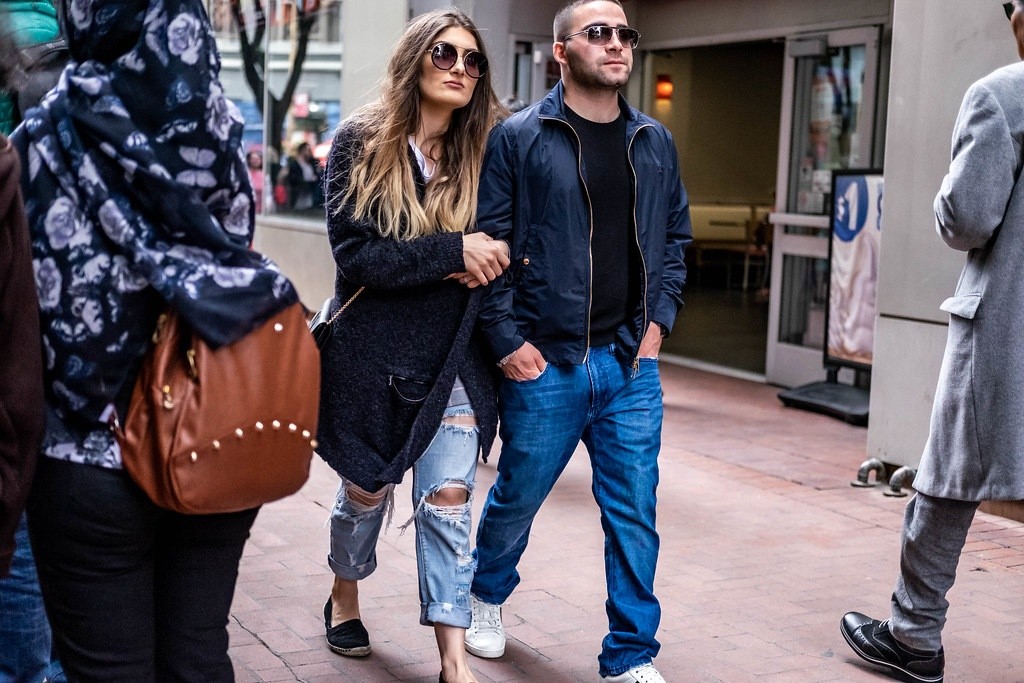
[309,297,335,353]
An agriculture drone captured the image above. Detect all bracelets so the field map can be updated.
[652,321,668,338]
[496,350,516,368]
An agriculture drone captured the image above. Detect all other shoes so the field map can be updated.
[324,595,372,657]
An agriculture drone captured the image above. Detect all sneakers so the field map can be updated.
[602,661,668,683]
[464,596,507,659]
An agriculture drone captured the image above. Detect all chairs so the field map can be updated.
[741,198,775,292]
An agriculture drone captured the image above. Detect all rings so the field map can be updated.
[463,277,468,283]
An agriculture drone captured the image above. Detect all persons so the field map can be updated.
[839,0,1024,683]
[245,142,323,214]
[464,0,691,683]
[324,7,510,683]
[0,0,264,683]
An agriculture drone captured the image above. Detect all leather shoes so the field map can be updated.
[841,608,946,683]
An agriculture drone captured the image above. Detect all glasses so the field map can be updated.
[560,25,643,50]
[425,42,489,80]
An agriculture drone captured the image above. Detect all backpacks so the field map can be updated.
[112,270,322,518]
